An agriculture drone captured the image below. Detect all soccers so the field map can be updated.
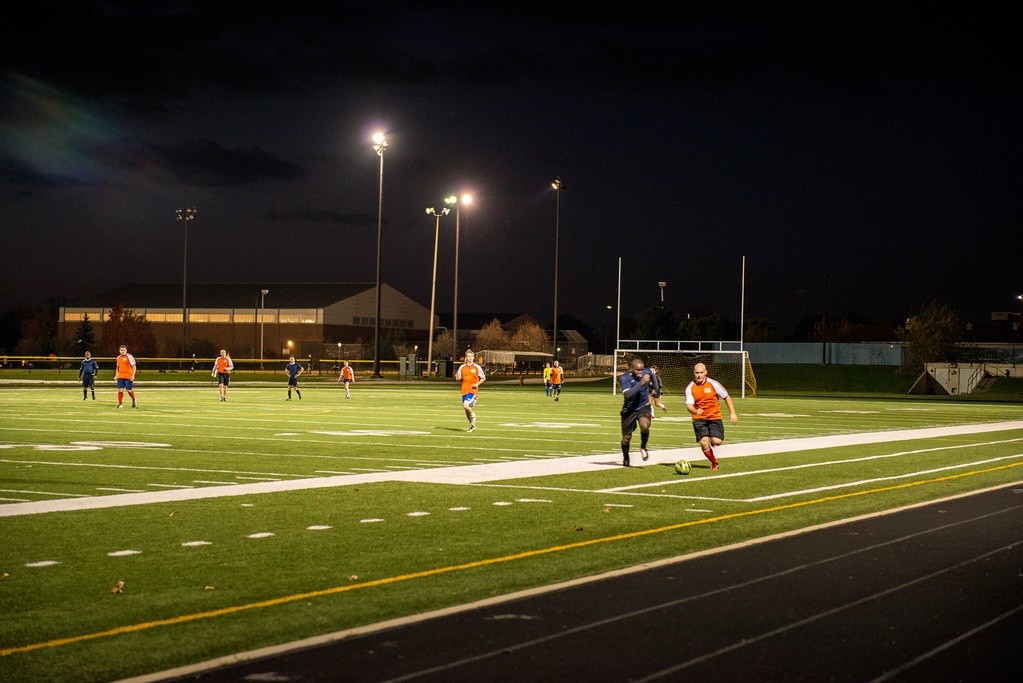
[675,460,691,474]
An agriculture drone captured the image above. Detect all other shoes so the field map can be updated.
[467,425,476,432]
[642,449,649,460]
[710,461,719,469]
[622,458,629,466]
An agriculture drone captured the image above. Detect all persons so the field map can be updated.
[338,361,354,398]
[330,358,339,374]
[213,350,233,401]
[620,360,658,466]
[285,356,303,401]
[191,353,197,371]
[78,352,98,400]
[114,346,137,408]
[543,361,564,401]
[456,351,485,432]
[686,363,737,470]
[649,367,667,418]
[307,354,311,374]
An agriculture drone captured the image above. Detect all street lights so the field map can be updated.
[369,129,388,379]
[656,280,669,306]
[551,177,563,365]
[174,206,196,363]
[444,193,474,362]
[604,305,612,354]
[421,205,451,376]
[260,288,270,370]
